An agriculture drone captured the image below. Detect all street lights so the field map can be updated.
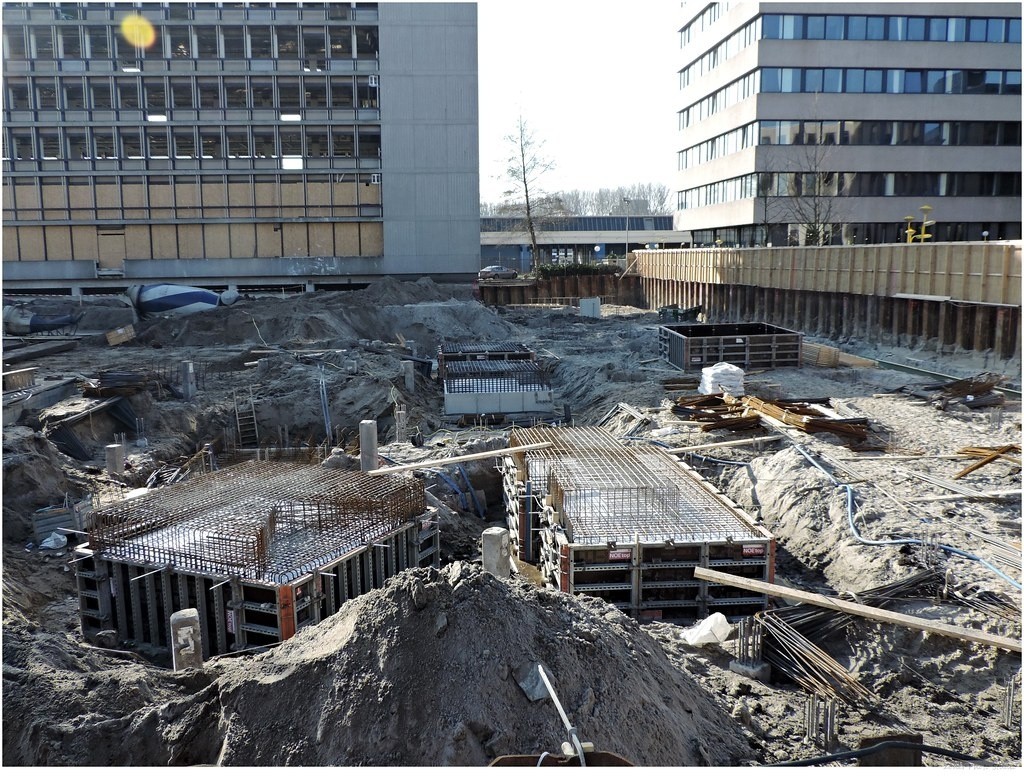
[622,198,631,273]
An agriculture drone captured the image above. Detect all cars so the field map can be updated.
[480,265,517,279]
